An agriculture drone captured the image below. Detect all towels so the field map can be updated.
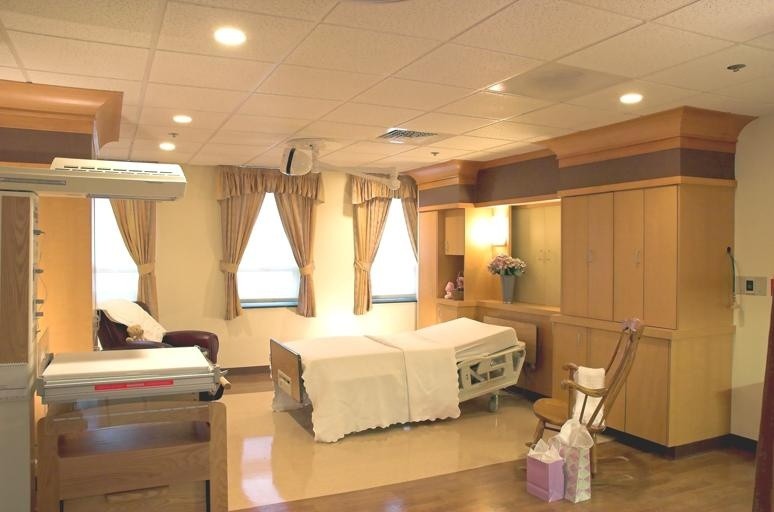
[569,361,610,430]
[95,300,168,343]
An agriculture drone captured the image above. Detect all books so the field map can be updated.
[36,345,217,403]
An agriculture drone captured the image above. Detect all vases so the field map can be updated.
[502,273,516,304]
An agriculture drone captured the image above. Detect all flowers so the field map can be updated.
[487,252,527,277]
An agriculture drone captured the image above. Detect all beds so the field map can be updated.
[269,317,526,427]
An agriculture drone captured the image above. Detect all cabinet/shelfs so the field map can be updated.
[552,322,733,449]
[415,209,480,332]
[560,184,735,329]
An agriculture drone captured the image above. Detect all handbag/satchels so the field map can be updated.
[550,437,591,503]
[526,450,565,502]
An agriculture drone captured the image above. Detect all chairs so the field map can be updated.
[524,316,646,475]
[92,300,220,363]
